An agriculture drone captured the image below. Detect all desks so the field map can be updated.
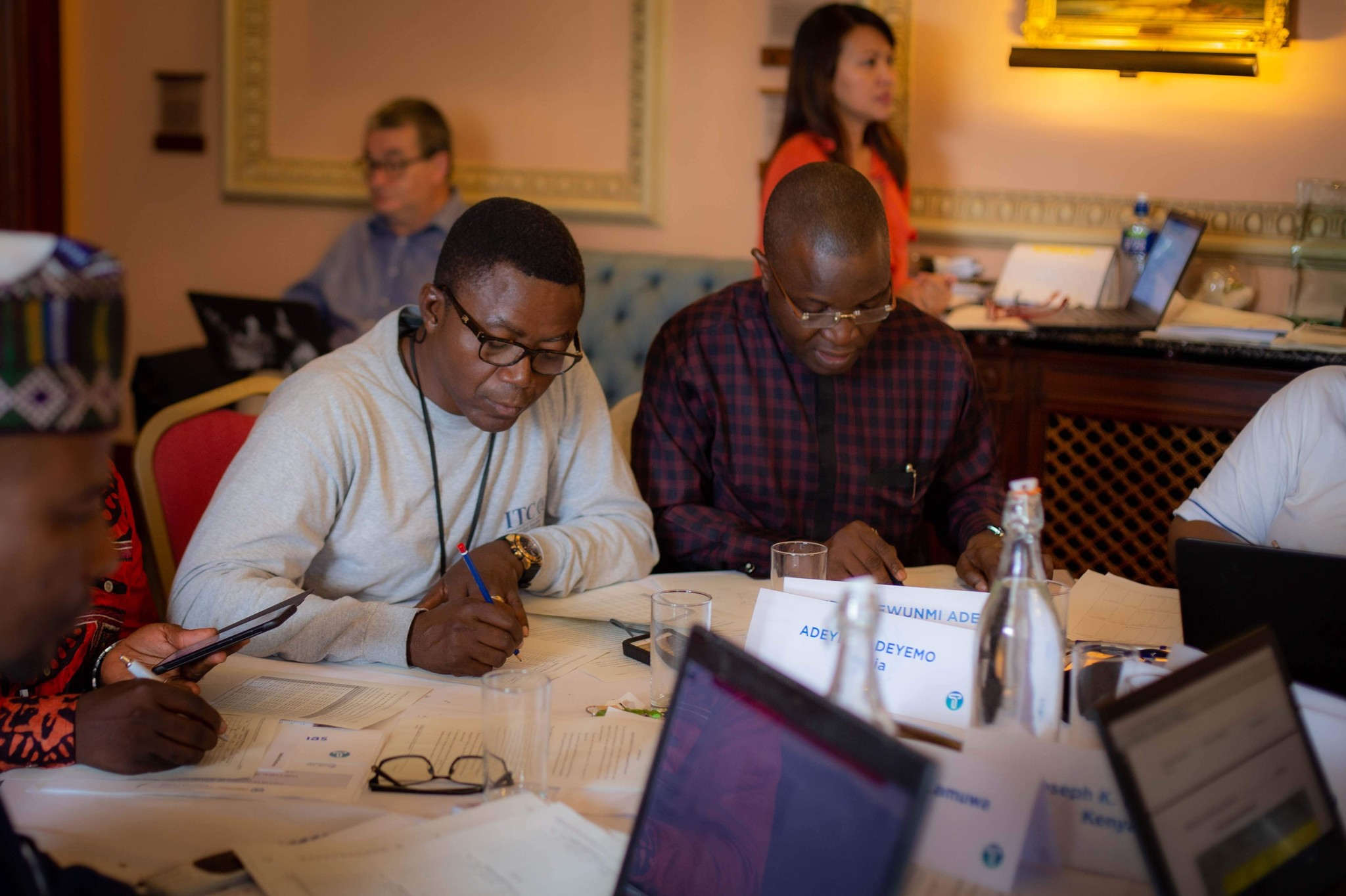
[2,571,1184,896]
[937,306,1346,599]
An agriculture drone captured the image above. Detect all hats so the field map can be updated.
[0,231,126,432]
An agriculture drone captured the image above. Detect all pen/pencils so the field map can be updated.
[1271,540,1281,549]
[119,654,230,742]
[869,528,905,586]
[454,544,524,662]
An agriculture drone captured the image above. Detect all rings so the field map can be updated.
[491,596,504,603]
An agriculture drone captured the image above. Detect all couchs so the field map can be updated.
[570,249,758,413]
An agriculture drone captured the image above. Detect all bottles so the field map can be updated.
[1119,203,1157,304]
[826,591,898,741]
[970,483,1065,741]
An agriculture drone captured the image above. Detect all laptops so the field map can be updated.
[608,624,938,896]
[1096,536,1346,896]
[185,292,334,376]
[1021,210,1208,334]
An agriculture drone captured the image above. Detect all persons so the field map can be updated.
[164,197,660,677]
[1169,365,1346,572]
[281,94,476,336]
[753,4,958,320]
[630,161,1053,593]
[0,232,140,896]
[0,455,250,775]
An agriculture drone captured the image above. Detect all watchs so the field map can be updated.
[494,533,543,589]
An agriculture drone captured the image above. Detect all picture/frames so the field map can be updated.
[213,3,667,225]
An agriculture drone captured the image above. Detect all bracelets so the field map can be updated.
[986,524,1005,535]
[92,638,126,689]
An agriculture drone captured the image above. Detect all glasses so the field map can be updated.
[765,252,893,329]
[431,279,583,376]
[368,751,515,796]
[360,152,435,180]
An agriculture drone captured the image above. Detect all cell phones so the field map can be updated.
[622,628,690,669]
[151,604,297,676]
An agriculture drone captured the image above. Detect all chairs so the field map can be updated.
[130,370,293,611]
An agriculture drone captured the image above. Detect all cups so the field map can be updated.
[1069,641,1140,730]
[479,670,553,799]
[649,590,712,716]
[770,542,828,594]
[1045,578,1070,639]
